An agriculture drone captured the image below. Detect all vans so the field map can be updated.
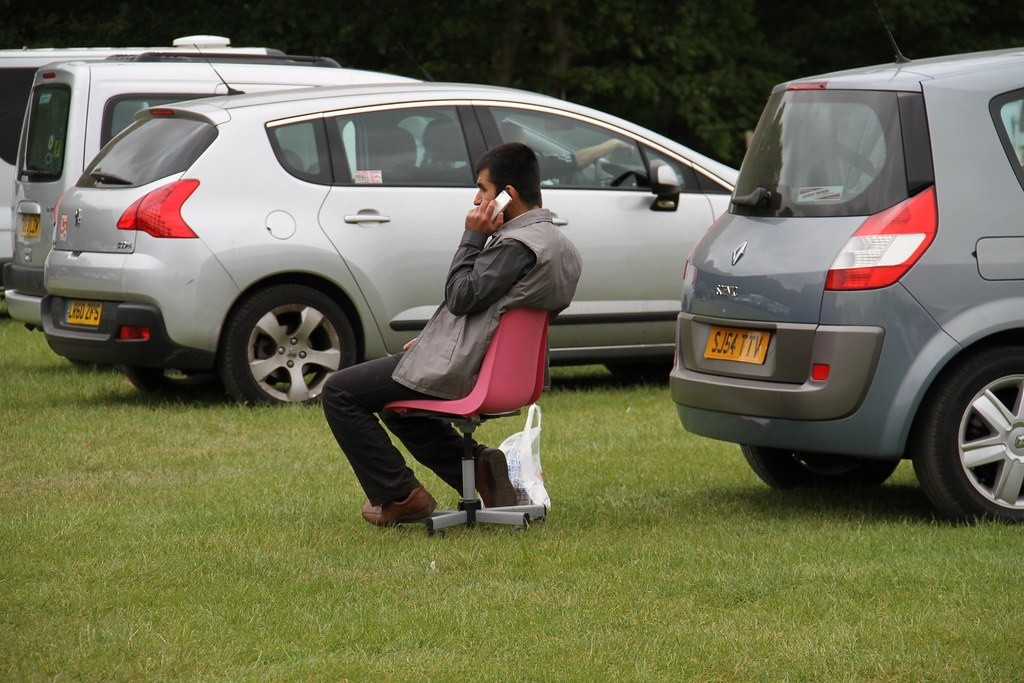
[0,33,427,333]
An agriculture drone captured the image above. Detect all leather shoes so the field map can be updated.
[360,478,436,526]
[476,446,516,510]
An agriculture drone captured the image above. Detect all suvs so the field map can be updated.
[670,43,1024,526]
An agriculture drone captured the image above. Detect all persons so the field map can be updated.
[321,144,584,528]
[501,122,635,183]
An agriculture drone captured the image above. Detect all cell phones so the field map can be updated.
[490,190,513,223]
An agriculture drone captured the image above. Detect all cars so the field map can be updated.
[39,82,740,410]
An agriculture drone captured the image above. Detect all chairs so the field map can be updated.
[382,127,424,179]
[384,306,552,535]
[424,115,469,181]
